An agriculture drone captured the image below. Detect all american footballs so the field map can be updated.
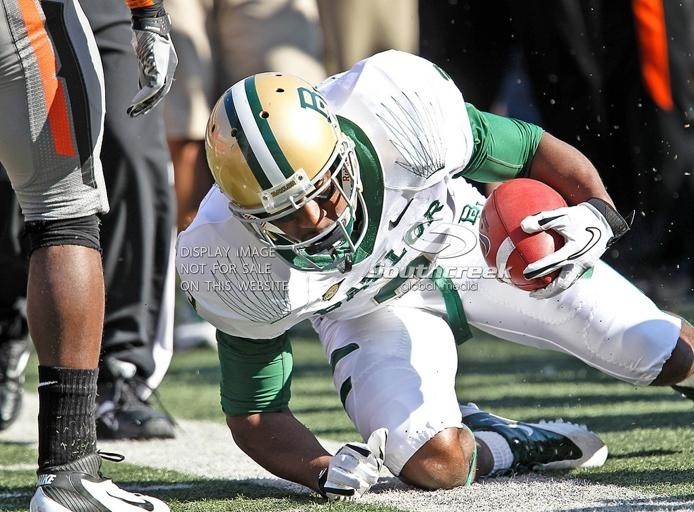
[479,178,568,291]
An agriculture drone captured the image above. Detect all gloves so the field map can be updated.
[125,0,179,119]
[316,427,389,502]
[517,197,636,301]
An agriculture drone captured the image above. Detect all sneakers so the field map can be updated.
[0,314,34,432]
[28,451,171,512]
[457,400,608,471]
[94,355,177,440]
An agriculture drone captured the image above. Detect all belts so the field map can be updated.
[428,266,473,346]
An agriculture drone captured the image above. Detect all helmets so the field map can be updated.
[203,71,347,225]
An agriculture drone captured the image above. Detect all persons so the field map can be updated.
[0,0,694,512]
